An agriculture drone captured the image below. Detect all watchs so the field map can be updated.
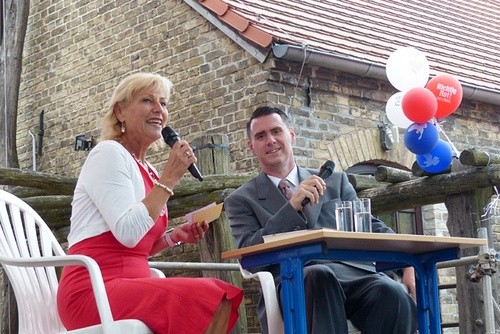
[163,228,182,248]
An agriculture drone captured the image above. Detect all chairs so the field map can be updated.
[0,189,166,334]
[237,257,362,334]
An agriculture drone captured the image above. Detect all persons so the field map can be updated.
[57,71,244,334]
[225,106,418,334]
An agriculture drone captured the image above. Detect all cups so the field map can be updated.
[353,198,372,233]
[335,201,353,232]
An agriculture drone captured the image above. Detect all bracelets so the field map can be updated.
[155,180,175,197]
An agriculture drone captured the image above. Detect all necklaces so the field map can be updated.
[120,140,155,181]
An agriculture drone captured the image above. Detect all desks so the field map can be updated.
[222,230,488,334]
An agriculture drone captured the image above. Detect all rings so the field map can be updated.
[193,234,201,238]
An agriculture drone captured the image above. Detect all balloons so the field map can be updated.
[416,139,453,173]
[385,46,430,91]
[386,91,415,128]
[404,121,439,155]
[426,73,463,118]
[402,87,438,123]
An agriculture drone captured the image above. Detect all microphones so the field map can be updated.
[161,126,203,181]
[304,160,335,204]
